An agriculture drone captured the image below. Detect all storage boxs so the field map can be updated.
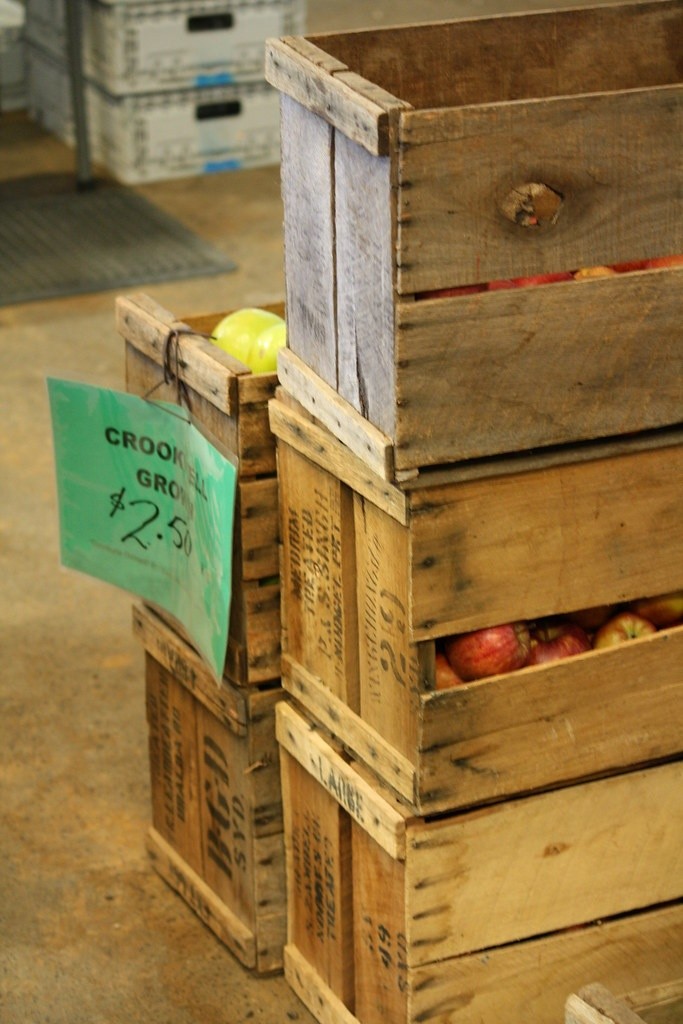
[19,0,311,100]
[275,699,683,1024]
[23,40,283,186]
[111,289,280,688]
[129,600,286,979]
[261,0,683,485]
[267,385,683,817]
[0,0,27,113]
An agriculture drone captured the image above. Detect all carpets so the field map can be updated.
[0,171,238,307]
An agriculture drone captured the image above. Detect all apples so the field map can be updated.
[431,590,683,691]
[209,307,287,377]
